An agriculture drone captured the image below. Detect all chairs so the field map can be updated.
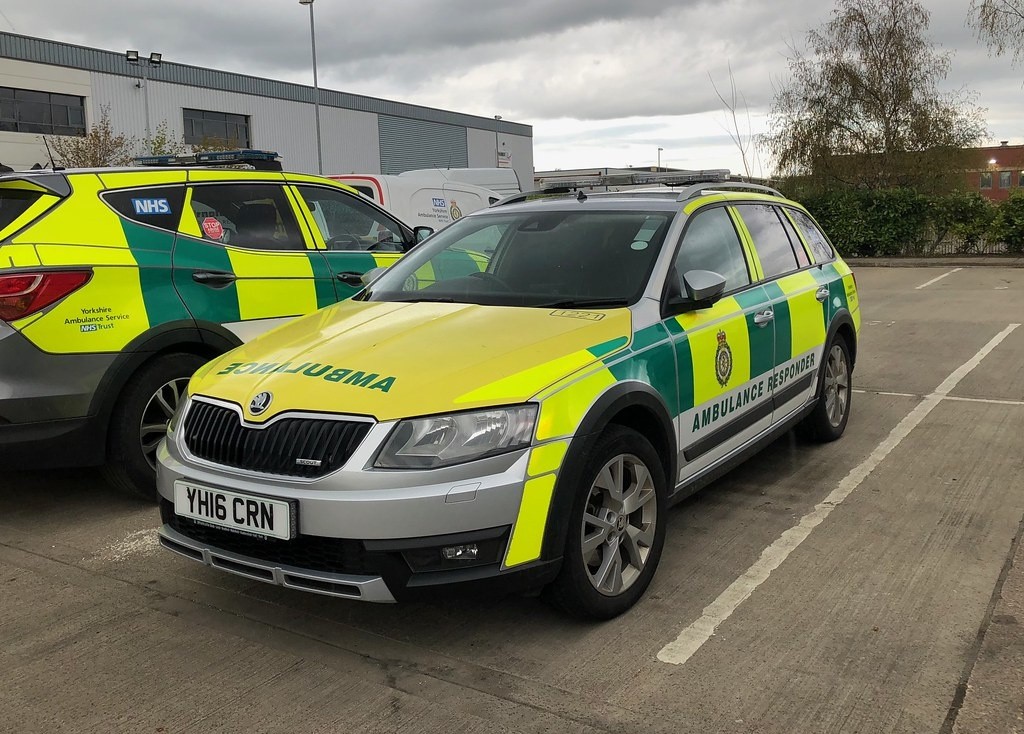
[234,204,283,249]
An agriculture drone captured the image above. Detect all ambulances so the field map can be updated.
[155,182,860,625]
[0,166,490,504]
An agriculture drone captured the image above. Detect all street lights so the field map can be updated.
[126,50,162,157]
[495,115,502,167]
[658,148,663,172]
